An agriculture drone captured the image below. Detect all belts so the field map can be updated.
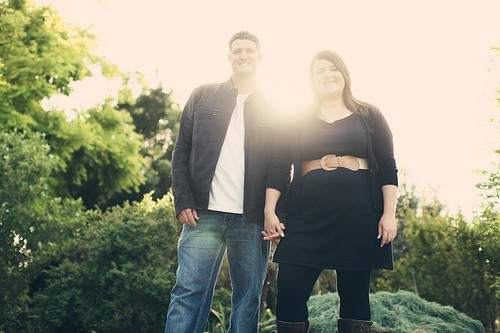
[300,154,369,178]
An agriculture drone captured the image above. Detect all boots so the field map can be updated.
[276,319,309,333]
[338,319,373,333]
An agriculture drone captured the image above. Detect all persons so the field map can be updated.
[261,49,399,333]
[165,30,291,333]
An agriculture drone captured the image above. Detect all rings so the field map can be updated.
[392,237,394,239]
